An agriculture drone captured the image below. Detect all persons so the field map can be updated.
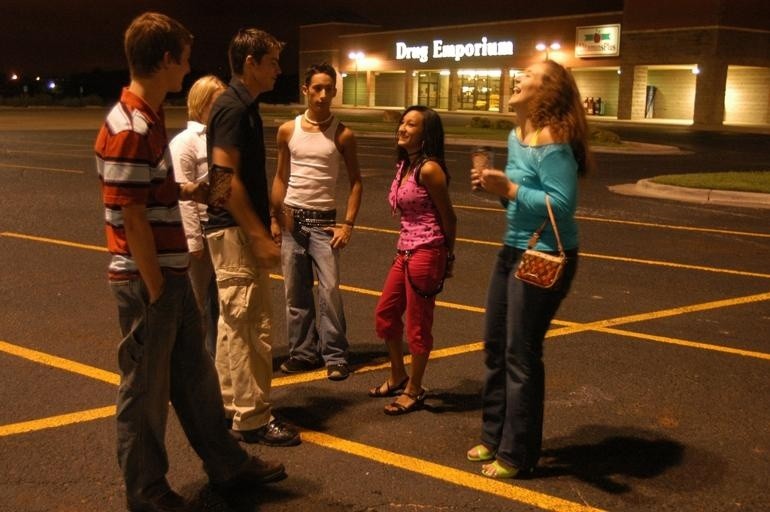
[366,105,456,417]
[92,13,287,511]
[167,75,226,369]
[206,26,300,446]
[270,64,363,382]
[465,59,596,480]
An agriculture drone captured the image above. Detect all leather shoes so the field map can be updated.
[225,410,302,447]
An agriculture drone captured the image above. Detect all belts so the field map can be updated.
[282,205,336,222]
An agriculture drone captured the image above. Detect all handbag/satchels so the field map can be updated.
[512,191,569,289]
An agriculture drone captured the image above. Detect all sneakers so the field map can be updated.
[280,356,325,373]
[125,485,197,512]
[208,456,291,490]
[326,363,350,380]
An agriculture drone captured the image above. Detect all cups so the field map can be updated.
[471,147,495,185]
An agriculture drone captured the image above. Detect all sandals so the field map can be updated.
[479,458,537,479]
[367,376,410,397]
[383,388,428,416]
[465,444,502,463]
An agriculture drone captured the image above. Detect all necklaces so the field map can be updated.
[302,107,335,127]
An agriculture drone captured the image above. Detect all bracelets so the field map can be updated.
[447,254,455,263]
[342,220,355,228]
